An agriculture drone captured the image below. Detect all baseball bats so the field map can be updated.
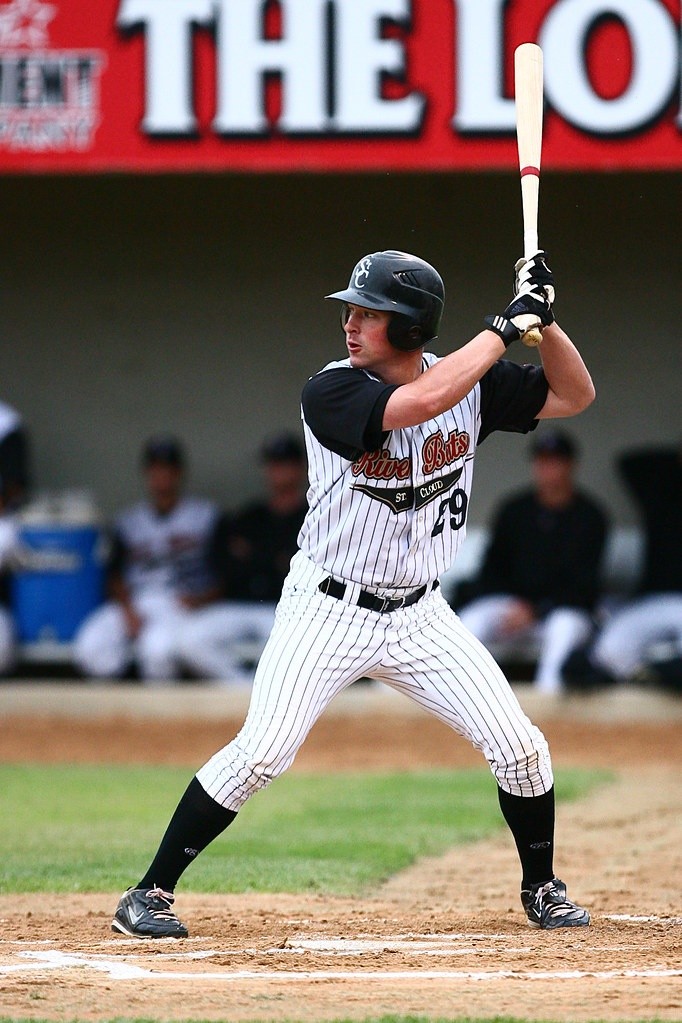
[513,43,544,348]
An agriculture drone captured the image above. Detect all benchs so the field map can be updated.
[17,527,676,664]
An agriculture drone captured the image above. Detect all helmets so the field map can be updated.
[323,250,444,352]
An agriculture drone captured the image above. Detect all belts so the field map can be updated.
[318,576,440,614]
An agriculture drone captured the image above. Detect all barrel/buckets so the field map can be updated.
[10,524,115,649]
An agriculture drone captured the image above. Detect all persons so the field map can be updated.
[0,400,32,677]
[108,248,597,940]
[593,442,681,691]
[178,429,309,679]
[76,428,217,676]
[451,427,613,693]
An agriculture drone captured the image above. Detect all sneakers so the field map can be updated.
[110,883,188,939]
[520,879,589,929]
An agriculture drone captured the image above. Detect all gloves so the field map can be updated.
[512,250,557,305]
[482,282,557,348]
